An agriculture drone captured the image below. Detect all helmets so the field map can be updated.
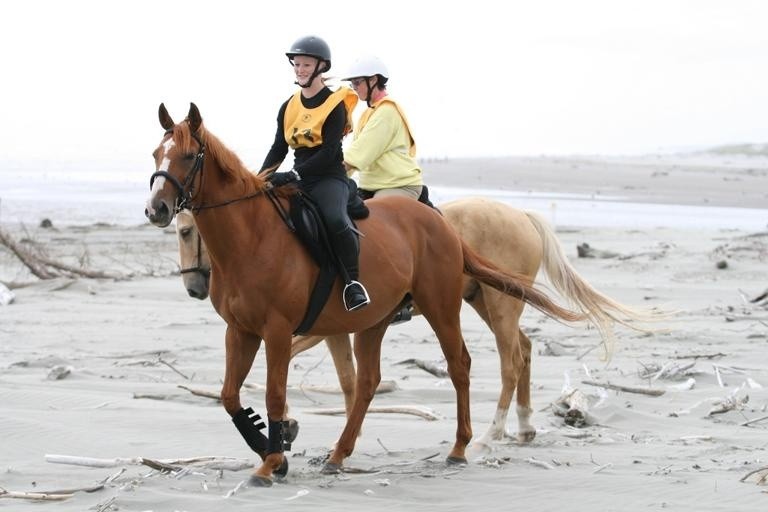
[340,52,391,83]
[285,35,332,71]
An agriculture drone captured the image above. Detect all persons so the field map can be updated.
[338,55,445,325]
[258,36,372,313]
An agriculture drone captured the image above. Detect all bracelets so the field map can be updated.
[291,167,303,182]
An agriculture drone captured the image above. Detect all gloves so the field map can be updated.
[264,170,296,188]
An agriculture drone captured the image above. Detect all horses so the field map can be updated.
[174,167,671,449]
[140,99,588,490]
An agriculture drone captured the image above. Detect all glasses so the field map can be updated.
[349,78,366,88]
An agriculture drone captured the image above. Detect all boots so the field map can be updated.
[330,220,367,311]
